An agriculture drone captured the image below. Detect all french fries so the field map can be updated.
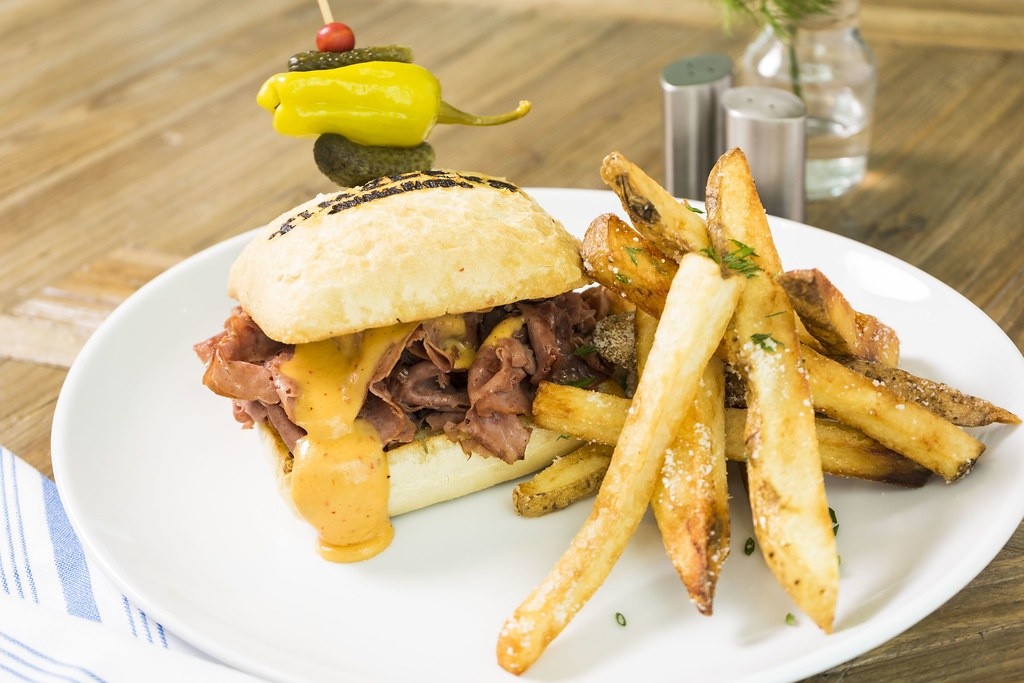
[496,150,985,677]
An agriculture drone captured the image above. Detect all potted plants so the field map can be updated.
[709,0,876,199]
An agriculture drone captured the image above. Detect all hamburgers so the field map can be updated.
[193,167,625,560]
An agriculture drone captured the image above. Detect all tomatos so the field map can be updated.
[316,21,355,52]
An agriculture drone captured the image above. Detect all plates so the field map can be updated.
[48,188,1024,683]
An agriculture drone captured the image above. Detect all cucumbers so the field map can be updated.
[313,132,436,188]
[288,45,413,72]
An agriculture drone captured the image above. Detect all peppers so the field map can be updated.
[256,62,530,149]
